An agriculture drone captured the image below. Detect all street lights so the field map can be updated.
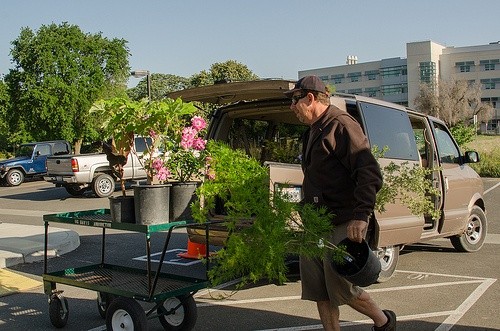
[129,69,153,105]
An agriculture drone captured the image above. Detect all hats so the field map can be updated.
[283,75,331,98]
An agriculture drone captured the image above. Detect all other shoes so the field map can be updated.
[371,310,397,331]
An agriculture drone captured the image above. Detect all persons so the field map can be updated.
[283,76,397,331]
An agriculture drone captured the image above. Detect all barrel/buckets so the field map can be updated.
[187,236,206,256]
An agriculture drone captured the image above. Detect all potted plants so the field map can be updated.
[90,98,134,224]
[190,140,381,287]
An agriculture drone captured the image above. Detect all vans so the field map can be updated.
[163,79,489,283]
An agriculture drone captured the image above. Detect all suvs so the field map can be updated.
[0,140,73,187]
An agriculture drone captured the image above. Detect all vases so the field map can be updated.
[171,180,201,220]
[134,181,169,224]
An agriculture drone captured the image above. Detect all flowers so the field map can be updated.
[169,113,216,181]
[147,130,170,184]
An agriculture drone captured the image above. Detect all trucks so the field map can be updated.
[45,133,208,199]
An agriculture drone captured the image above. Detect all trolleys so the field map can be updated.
[43,208,239,331]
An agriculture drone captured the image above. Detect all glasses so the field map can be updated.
[291,94,307,105]
